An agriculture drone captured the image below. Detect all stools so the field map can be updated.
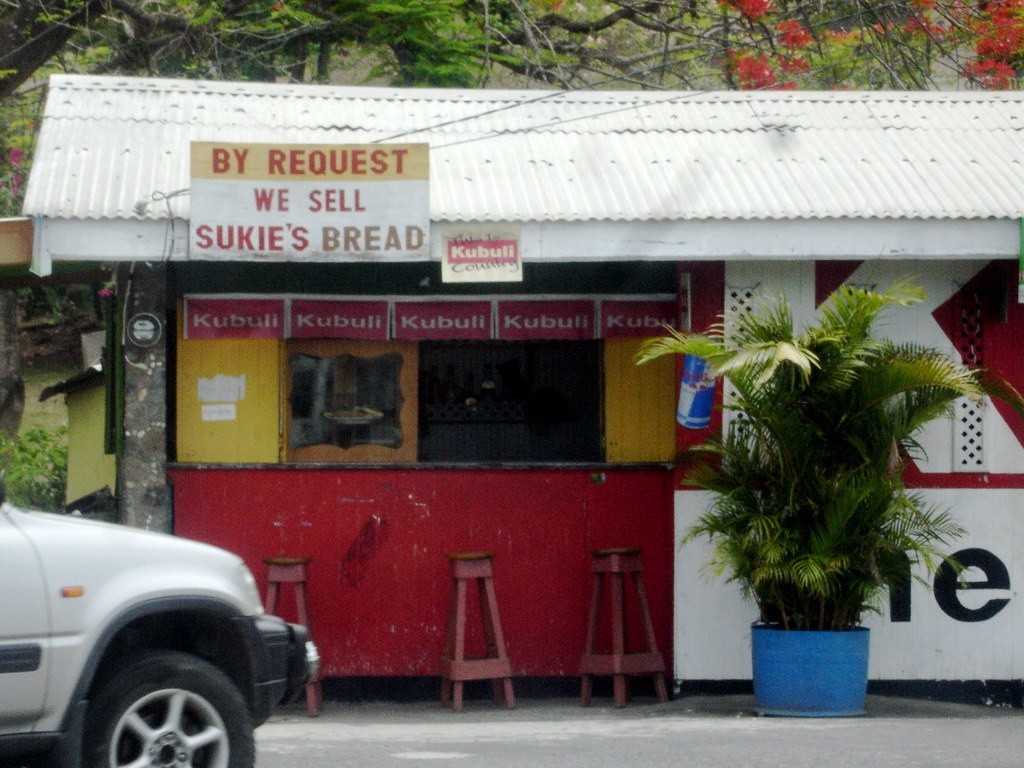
[579,547,669,707]
[262,556,324,718]
[438,551,517,712]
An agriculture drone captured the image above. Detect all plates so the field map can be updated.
[323,412,384,425]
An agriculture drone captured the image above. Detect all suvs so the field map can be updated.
[0,476,320,768]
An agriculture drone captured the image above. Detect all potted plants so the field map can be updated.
[632,275,1024,717]
